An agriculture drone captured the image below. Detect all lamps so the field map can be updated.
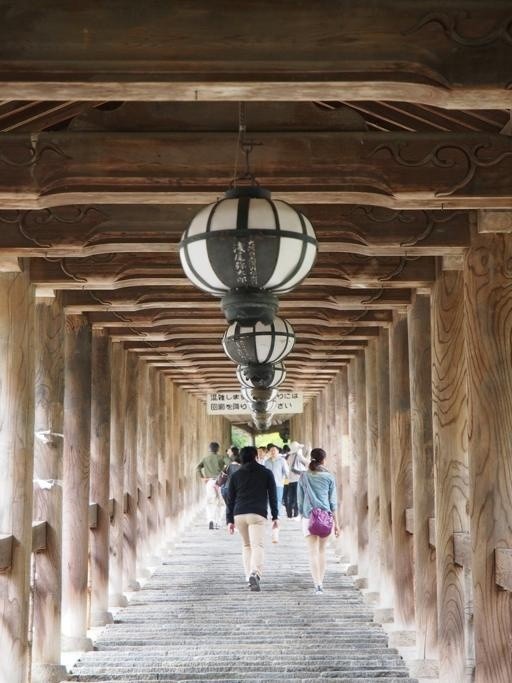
[235,384,280,432]
[232,360,293,400]
[173,146,317,320]
[216,315,300,380]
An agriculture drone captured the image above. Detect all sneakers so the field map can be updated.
[315,584,324,595]
[249,572,261,590]
[208,520,214,529]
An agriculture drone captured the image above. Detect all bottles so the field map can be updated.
[272,521,279,543]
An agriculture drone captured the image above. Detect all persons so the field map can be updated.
[195,440,311,531]
[226,446,279,593]
[295,447,339,591]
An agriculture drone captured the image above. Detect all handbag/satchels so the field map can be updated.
[215,464,230,486]
[291,454,307,474]
[308,507,333,536]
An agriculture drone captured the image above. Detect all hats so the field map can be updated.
[287,440,304,454]
